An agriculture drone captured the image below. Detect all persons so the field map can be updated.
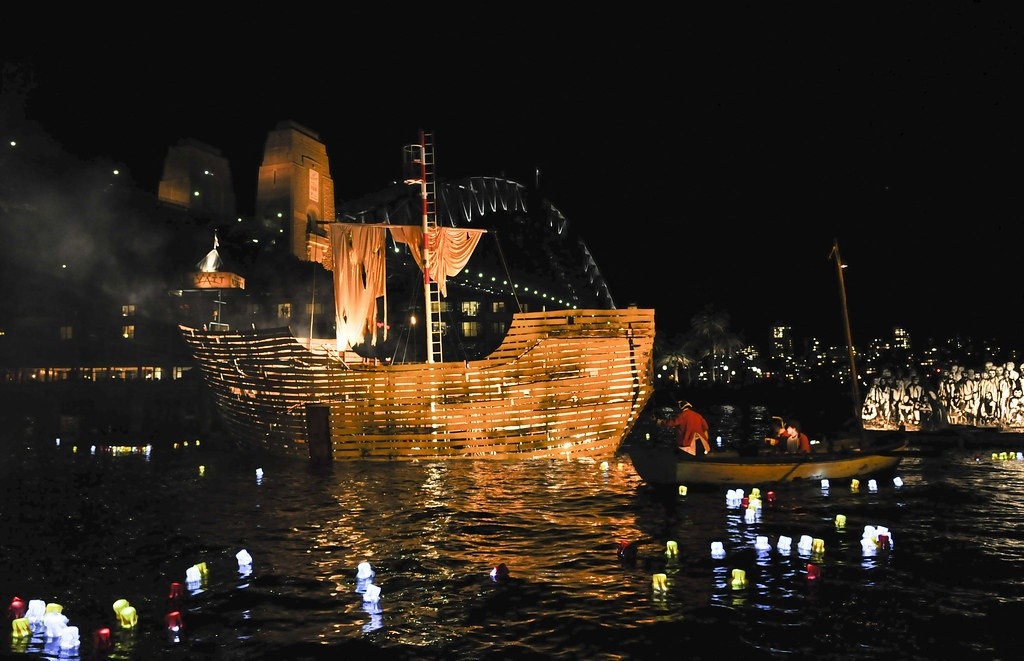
[650,399,710,459]
[860,361,1024,427]
[764,414,810,455]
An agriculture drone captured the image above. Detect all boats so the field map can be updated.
[181,123,662,465]
[628,239,900,488]
[891,444,924,467]
